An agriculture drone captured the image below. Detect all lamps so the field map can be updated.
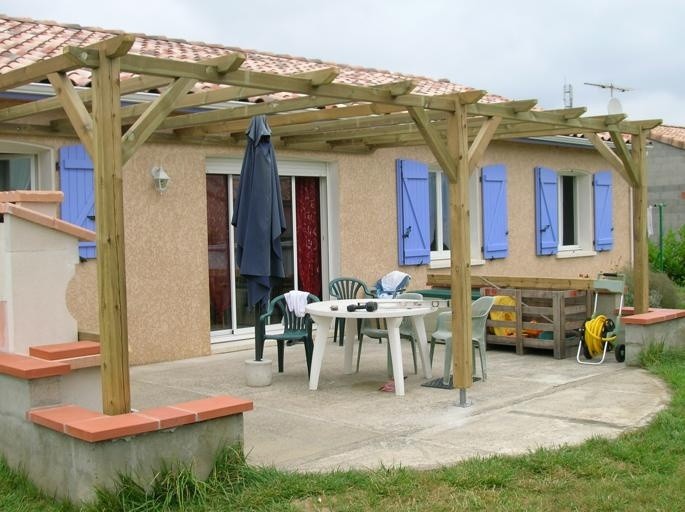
[152,160,171,195]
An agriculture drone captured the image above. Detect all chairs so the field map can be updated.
[255,270,494,397]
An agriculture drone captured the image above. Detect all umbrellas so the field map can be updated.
[228,113,289,362]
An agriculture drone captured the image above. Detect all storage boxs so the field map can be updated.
[479,286,591,359]
